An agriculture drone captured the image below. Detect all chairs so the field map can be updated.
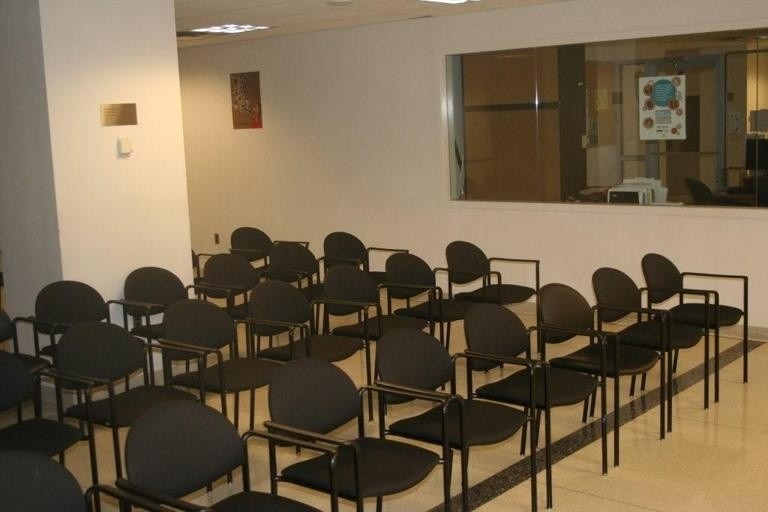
[687,166,767,206]
